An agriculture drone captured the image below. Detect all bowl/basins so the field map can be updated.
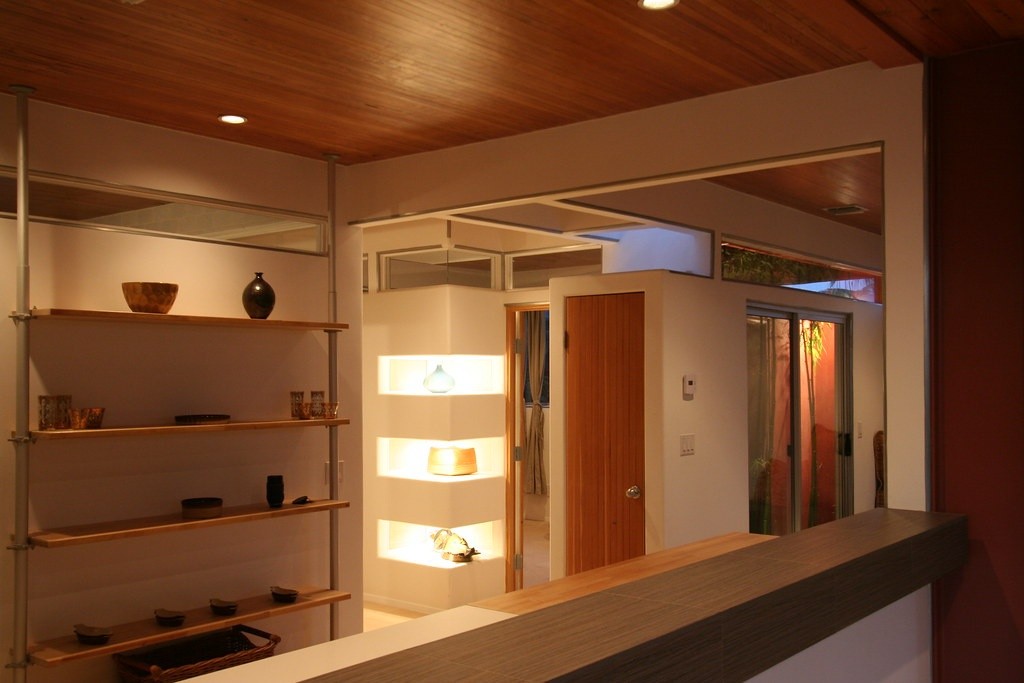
[299,402,339,419]
[121,282,179,314]
[66,407,105,430]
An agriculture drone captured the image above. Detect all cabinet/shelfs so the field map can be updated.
[6,91,352,683]
[362,282,507,615]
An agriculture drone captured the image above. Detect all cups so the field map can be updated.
[266,474,285,509]
[310,390,325,402]
[38,394,71,431]
[290,390,304,417]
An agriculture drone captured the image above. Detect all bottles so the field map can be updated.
[242,272,276,319]
[423,364,455,393]
